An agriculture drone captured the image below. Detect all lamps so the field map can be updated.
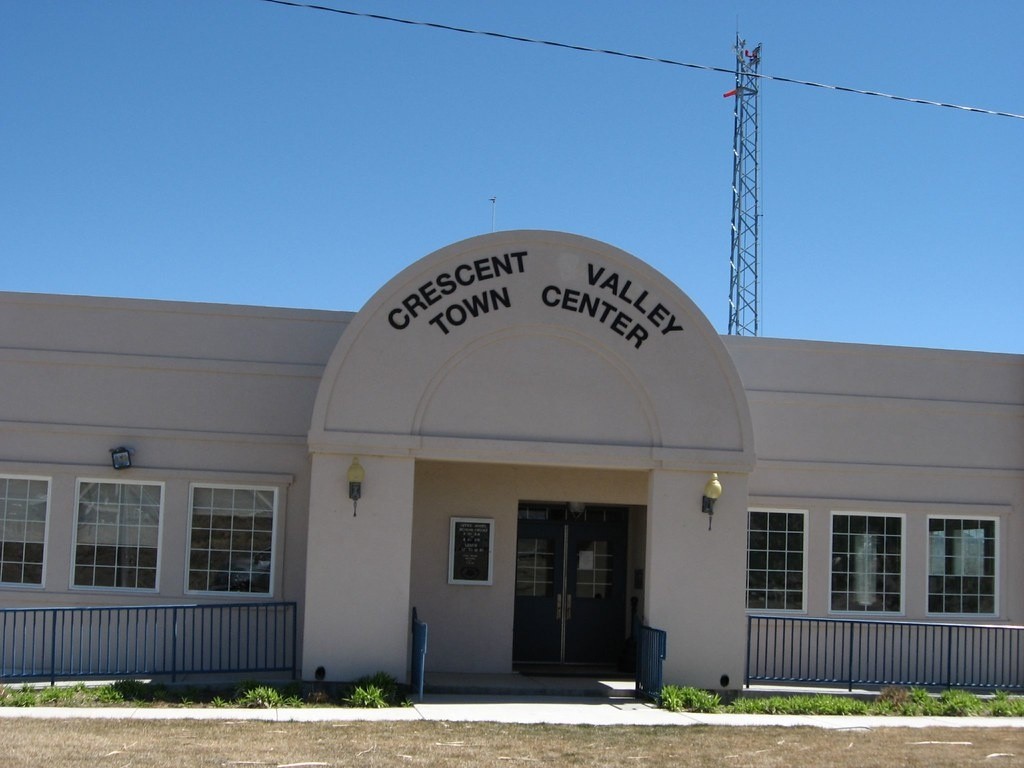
[701,472,722,533]
[347,456,364,516]
[109,446,131,470]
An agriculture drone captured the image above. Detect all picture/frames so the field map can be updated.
[448,517,494,586]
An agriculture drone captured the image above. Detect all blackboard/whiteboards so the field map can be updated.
[448,517,495,586]
